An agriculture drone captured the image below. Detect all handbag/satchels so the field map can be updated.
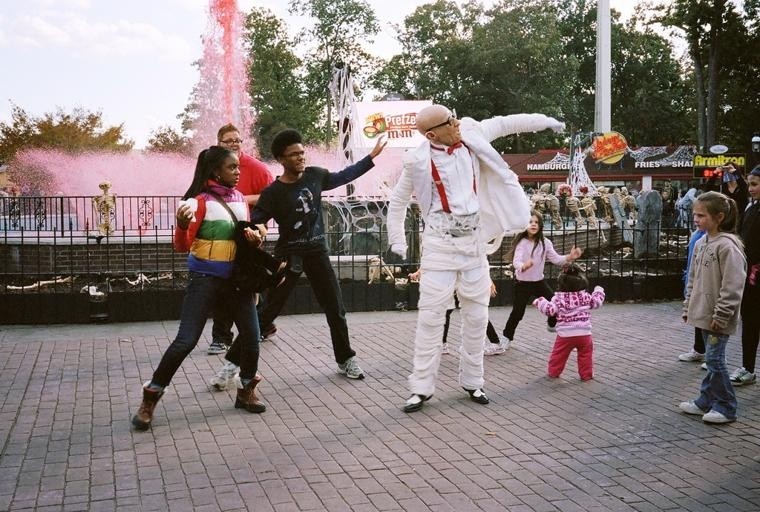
[231,246,278,295]
[282,239,306,275]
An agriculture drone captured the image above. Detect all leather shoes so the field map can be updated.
[404,387,434,413]
[461,380,490,404]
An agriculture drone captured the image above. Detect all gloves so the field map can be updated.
[389,244,410,262]
[547,113,568,138]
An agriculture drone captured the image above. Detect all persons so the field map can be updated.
[386,104,567,412]
[705,162,749,213]
[209,123,277,357]
[678,201,712,371]
[406,260,506,356]
[125,147,266,430]
[676,189,747,424]
[499,210,583,348]
[210,133,390,393]
[726,165,760,386]
[531,264,606,381]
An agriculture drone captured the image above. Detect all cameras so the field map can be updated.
[722,166,729,170]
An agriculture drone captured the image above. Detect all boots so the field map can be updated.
[132,382,162,427]
[235,374,266,413]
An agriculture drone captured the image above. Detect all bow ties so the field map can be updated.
[447,142,462,155]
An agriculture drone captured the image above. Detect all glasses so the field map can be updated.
[280,151,310,160]
[218,137,245,145]
[425,107,457,133]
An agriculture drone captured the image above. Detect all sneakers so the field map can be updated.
[701,360,710,369]
[442,343,449,356]
[259,324,278,342]
[729,366,757,386]
[211,360,239,392]
[678,349,708,361]
[483,342,506,356]
[702,406,739,424]
[336,355,366,381]
[545,323,556,333]
[500,332,511,350]
[207,335,233,353]
[678,396,709,415]
